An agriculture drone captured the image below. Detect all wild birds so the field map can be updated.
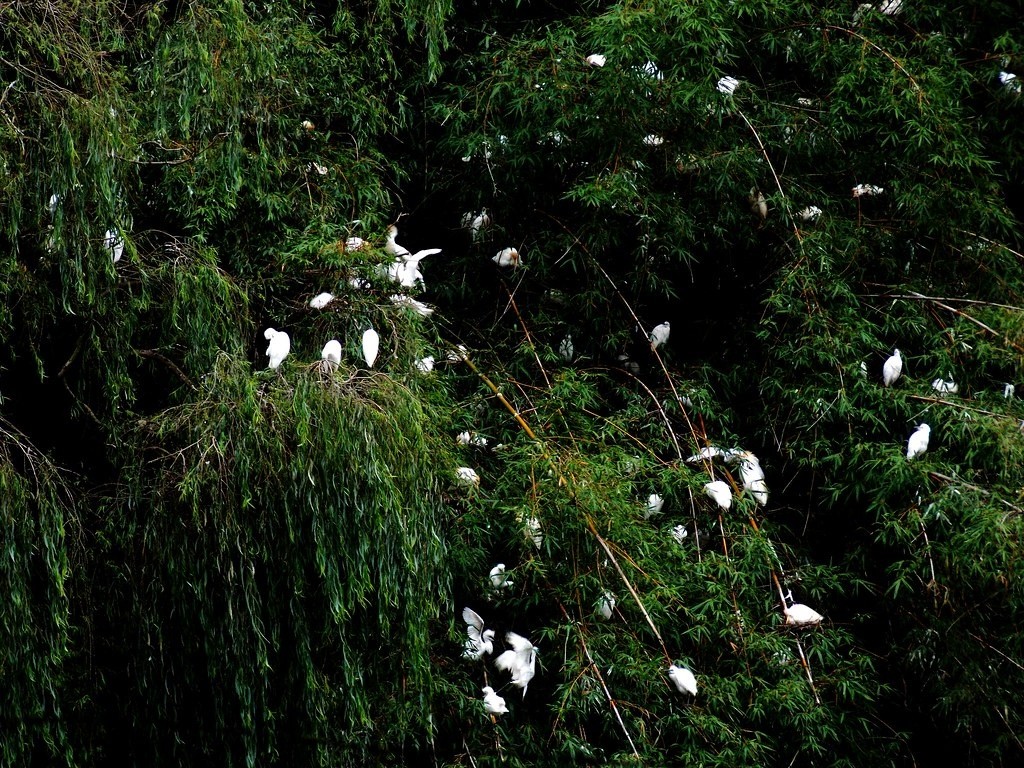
[491,247,524,269]
[928,372,959,396]
[852,0,903,28]
[668,666,699,696]
[453,428,545,720]
[781,603,824,628]
[793,205,823,221]
[881,348,905,388]
[460,53,739,150]
[648,321,672,352]
[263,326,470,376]
[906,423,931,463]
[46,182,127,267]
[998,71,1022,93]
[593,590,619,618]
[309,217,443,322]
[749,187,768,222]
[461,207,490,242]
[852,183,884,198]
[645,444,769,546]
